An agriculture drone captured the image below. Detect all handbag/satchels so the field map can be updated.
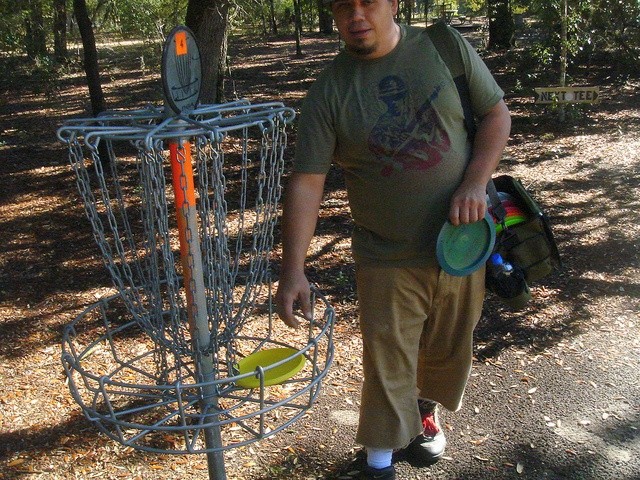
[486,174,561,311]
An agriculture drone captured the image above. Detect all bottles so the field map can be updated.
[488,253,510,276]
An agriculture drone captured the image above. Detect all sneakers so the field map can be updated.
[410,410,445,462]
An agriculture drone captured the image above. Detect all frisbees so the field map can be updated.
[486,191,528,234]
[233,348,305,389]
[436,213,498,277]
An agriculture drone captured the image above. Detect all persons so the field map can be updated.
[274,2,513,480]
